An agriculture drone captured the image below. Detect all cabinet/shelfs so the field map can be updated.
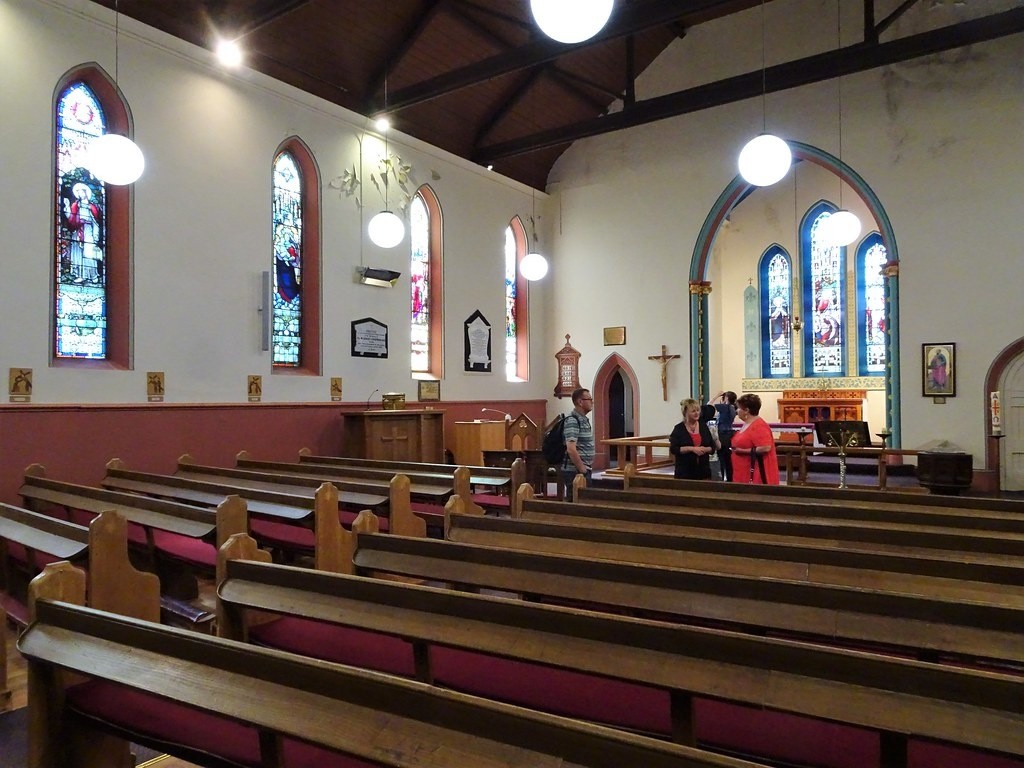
[777,397,867,423]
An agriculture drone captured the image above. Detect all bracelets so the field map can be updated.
[713,397,716,401]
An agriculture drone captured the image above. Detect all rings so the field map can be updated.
[701,449,703,451]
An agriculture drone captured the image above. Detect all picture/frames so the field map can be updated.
[921,342,957,398]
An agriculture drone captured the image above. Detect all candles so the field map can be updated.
[882,428,886,434]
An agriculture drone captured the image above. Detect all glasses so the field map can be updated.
[581,398,593,402]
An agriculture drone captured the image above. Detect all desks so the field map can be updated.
[455,420,528,489]
[732,422,815,482]
[341,408,447,463]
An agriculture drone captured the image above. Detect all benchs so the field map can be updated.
[510,483,1024,565]
[297,448,526,519]
[216,533,1024,768]
[444,494,1024,587]
[172,453,427,538]
[14,561,768,768]
[571,474,1024,540]
[233,450,486,516]
[0,502,161,624]
[22,463,253,636]
[98,458,353,578]
[350,509,1024,674]
[624,463,1024,535]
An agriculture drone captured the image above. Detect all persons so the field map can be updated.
[560,389,596,503]
[700,404,723,481]
[63,181,101,284]
[730,394,780,486]
[706,390,735,482]
[669,398,716,481]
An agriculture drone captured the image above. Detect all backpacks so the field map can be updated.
[542,415,581,462]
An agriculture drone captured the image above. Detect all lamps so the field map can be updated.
[367,78,405,248]
[819,0,861,247]
[85,0,145,186]
[790,317,805,334]
[738,0,792,186]
[519,83,548,281]
